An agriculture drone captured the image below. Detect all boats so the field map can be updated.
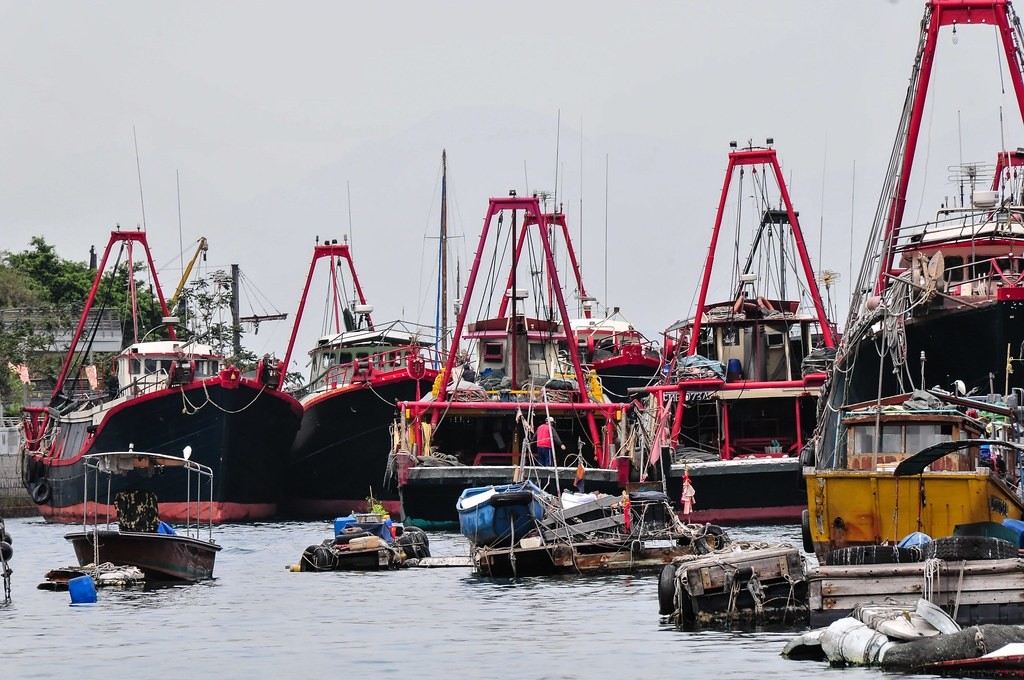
[389,189,632,529]
[800,348,1023,564]
[38,441,221,588]
[628,139,844,524]
[898,151,1024,315]
[16,221,304,525]
[418,148,470,369]
[499,211,663,405]
[796,0,1024,465]
[285,484,431,571]
[782,534,1024,680]
[455,479,551,549]
[471,490,793,581]
[707,207,843,382]
[277,233,442,519]
[0,519,15,609]
[656,537,810,630]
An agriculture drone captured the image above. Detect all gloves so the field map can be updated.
[561,445,566,450]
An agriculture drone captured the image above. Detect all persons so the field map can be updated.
[530,416,567,467]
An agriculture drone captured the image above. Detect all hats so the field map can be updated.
[545,417,556,424]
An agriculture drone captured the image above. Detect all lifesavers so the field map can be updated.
[657,563,677,616]
[693,523,730,557]
[400,526,431,560]
[299,538,338,573]
[800,508,816,555]
[86,424,99,433]
[31,480,52,506]
